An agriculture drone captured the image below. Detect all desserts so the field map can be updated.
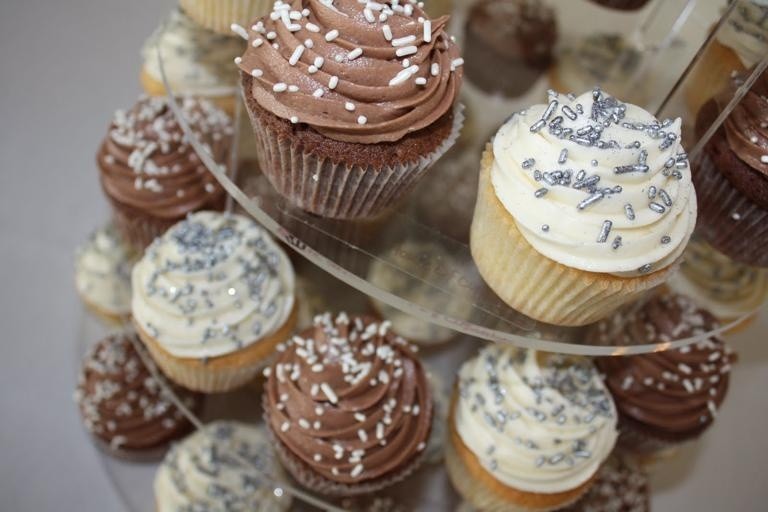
[75,0,768,512]
